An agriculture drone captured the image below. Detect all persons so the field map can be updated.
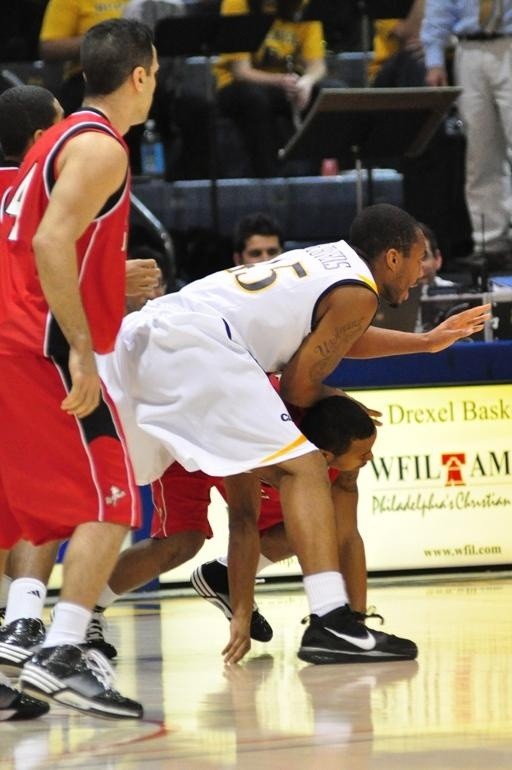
[85,370,376,663]
[0,0,512,337]
[49,200,493,661]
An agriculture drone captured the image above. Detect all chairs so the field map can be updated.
[127,51,423,332]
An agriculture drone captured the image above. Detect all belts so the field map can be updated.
[457,32,504,42]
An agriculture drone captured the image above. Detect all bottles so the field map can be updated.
[138,118,168,180]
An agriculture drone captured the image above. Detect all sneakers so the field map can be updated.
[1,611,50,719]
[290,603,420,665]
[17,642,147,725]
[189,554,278,643]
[49,602,120,665]
